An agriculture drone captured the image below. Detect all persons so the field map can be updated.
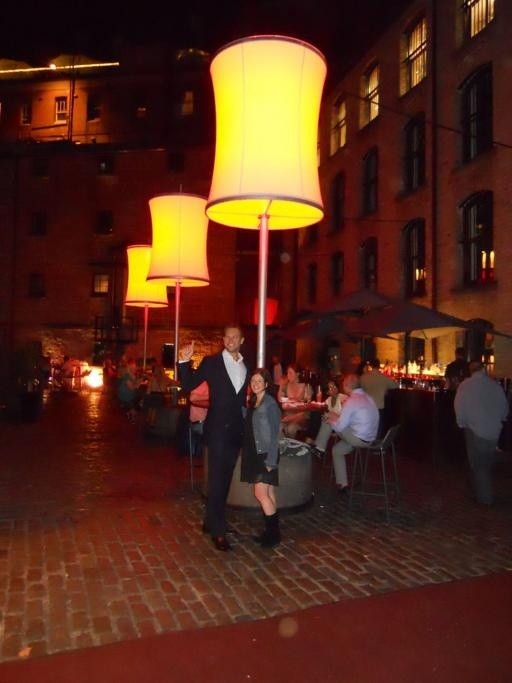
[119,363,140,410]
[309,373,379,495]
[453,359,509,511]
[445,347,471,394]
[178,326,251,551]
[241,368,281,548]
[189,380,209,434]
[269,354,396,447]
[60,355,74,393]
[147,362,167,395]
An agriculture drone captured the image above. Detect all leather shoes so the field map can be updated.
[340,486,351,496]
[213,535,229,550]
[307,444,325,458]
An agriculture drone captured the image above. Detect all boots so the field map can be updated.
[255,513,281,547]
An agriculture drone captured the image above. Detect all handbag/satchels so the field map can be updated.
[280,437,311,456]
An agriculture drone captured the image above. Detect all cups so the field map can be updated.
[304,385,312,403]
[316,392,322,402]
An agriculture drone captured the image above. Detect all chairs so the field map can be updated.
[347,423,403,498]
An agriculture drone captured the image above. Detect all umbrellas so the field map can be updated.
[347,300,511,383]
